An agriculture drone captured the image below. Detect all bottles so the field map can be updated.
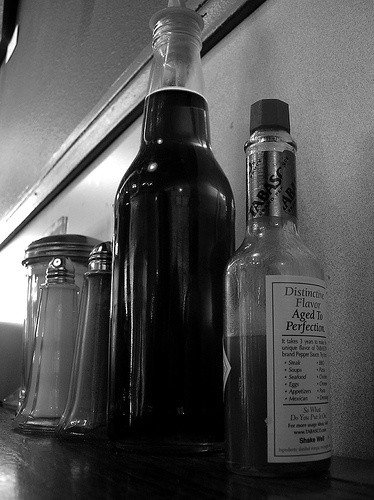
[110,6,235,452]
[56,243,116,440]
[220,98,330,478]
[11,252,79,434]
